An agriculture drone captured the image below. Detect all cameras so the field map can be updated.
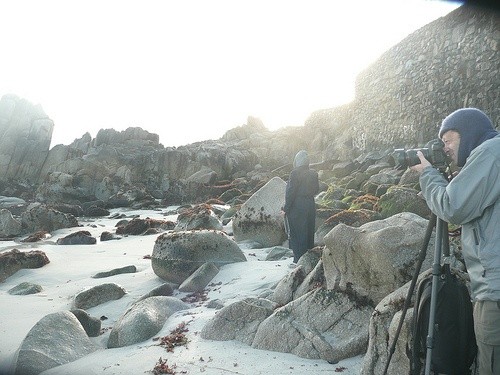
[394,140,446,172]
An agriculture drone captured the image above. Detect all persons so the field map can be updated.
[279,149,321,264]
[413,107,500,375]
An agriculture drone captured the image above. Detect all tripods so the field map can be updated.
[382,168,449,375]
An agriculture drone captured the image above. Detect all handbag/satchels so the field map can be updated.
[406,264,476,375]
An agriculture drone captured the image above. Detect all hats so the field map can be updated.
[293,150,309,168]
[439,107,497,167]
